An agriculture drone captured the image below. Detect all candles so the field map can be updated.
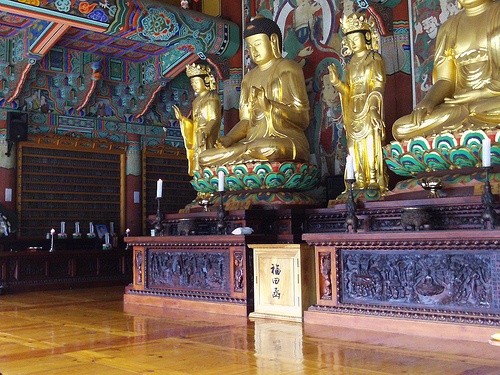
[218,171,224,192]
[346,154,354,179]
[157,178,162,198]
[482,138,490,167]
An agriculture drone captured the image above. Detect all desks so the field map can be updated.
[0,248,133,295]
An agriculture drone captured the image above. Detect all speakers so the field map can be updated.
[7,112,28,141]
